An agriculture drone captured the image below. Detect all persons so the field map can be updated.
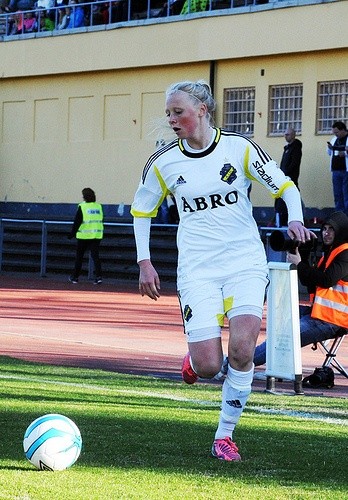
[131,80,317,461]
[327,122,348,215]
[214,211,348,381]
[0,0,273,35]
[274,129,302,228]
[67,187,104,285]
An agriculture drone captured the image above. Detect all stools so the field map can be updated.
[311,334,348,382]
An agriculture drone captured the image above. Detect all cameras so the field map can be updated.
[309,366,334,387]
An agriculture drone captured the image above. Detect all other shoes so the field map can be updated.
[213,366,225,380]
[68,275,78,284]
[94,277,103,285]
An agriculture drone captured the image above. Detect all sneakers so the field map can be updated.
[181,352,198,384]
[211,436,241,462]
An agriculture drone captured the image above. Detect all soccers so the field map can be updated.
[22,413,83,472]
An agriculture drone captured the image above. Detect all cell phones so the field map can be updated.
[327,141,330,144]
